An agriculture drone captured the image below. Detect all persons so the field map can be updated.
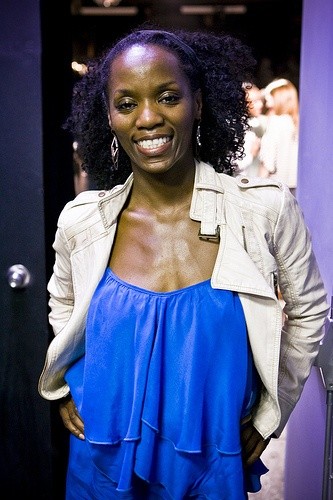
[38,26,328,499]
[240,73,298,185]
